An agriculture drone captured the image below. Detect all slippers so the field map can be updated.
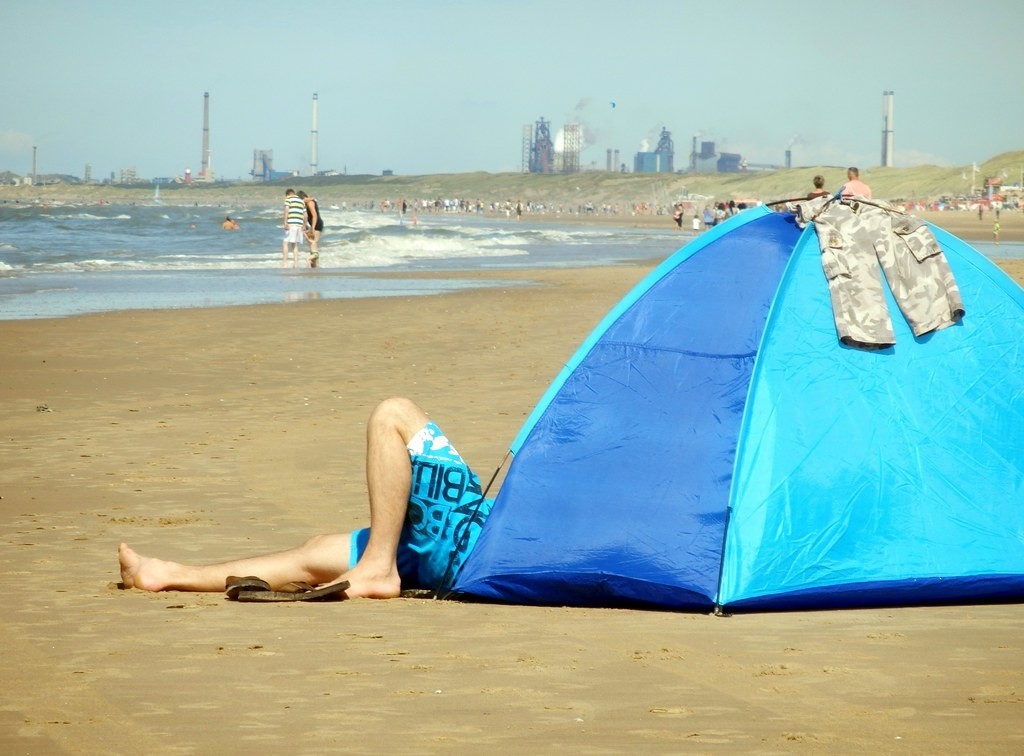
[225,573,351,601]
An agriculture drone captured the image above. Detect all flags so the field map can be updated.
[974,163,980,173]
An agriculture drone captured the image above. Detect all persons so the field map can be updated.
[979,202,983,220]
[222,218,240,230]
[993,219,1000,243]
[379,198,747,235]
[995,203,1000,218]
[807,176,830,199]
[283,189,323,267]
[838,167,871,199]
[117,398,497,600]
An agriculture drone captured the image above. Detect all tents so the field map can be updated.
[439,193,1024,615]
